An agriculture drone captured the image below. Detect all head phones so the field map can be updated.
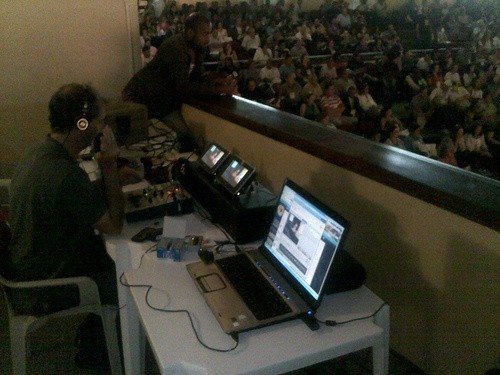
[74,85,91,132]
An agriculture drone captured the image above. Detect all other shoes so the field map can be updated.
[76,340,110,368]
[75,313,104,338]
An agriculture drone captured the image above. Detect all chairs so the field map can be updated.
[0,179,123,375]
[148,10,500,148]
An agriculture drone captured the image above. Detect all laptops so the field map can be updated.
[186,177,350,336]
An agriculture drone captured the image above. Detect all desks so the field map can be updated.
[69,147,390,375]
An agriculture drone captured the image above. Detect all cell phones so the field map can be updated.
[131,228,154,241]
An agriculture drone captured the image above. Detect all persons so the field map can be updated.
[117,14,237,165]
[0,83,145,375]
[137,0,500,182]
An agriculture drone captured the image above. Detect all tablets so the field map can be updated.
[197,142,232,176]
[217,155,255,195]
